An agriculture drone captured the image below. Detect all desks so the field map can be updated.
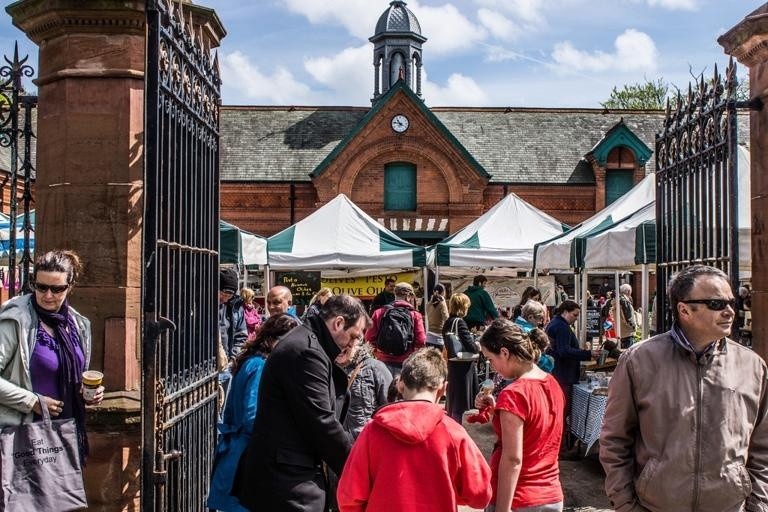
[570,380,609,458]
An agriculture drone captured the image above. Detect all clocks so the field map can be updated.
[390,114,409,133]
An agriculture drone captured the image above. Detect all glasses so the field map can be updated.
[31,281,69,294]
[679,299,737,311]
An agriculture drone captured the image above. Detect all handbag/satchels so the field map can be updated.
[445,332,480,363]
[0,418,88,512]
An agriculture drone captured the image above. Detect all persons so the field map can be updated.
[204,266,636,512]
[649,296,657,337]
[599,265,768,512]
[0,250,104,470]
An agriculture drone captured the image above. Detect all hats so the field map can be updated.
[394,282,415,296]
[220,270,237,293]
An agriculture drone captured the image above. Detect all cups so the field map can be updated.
[596,350,606,367]
[82,370,104,402]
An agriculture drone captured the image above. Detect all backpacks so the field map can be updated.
[377,305,416,356]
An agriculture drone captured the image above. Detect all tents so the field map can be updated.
[425,188,630,334]
[220,218,269,316]
[255,188,427,334]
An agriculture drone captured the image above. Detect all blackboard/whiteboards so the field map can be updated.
[586,309,601,337]
[275,271,321,305]
